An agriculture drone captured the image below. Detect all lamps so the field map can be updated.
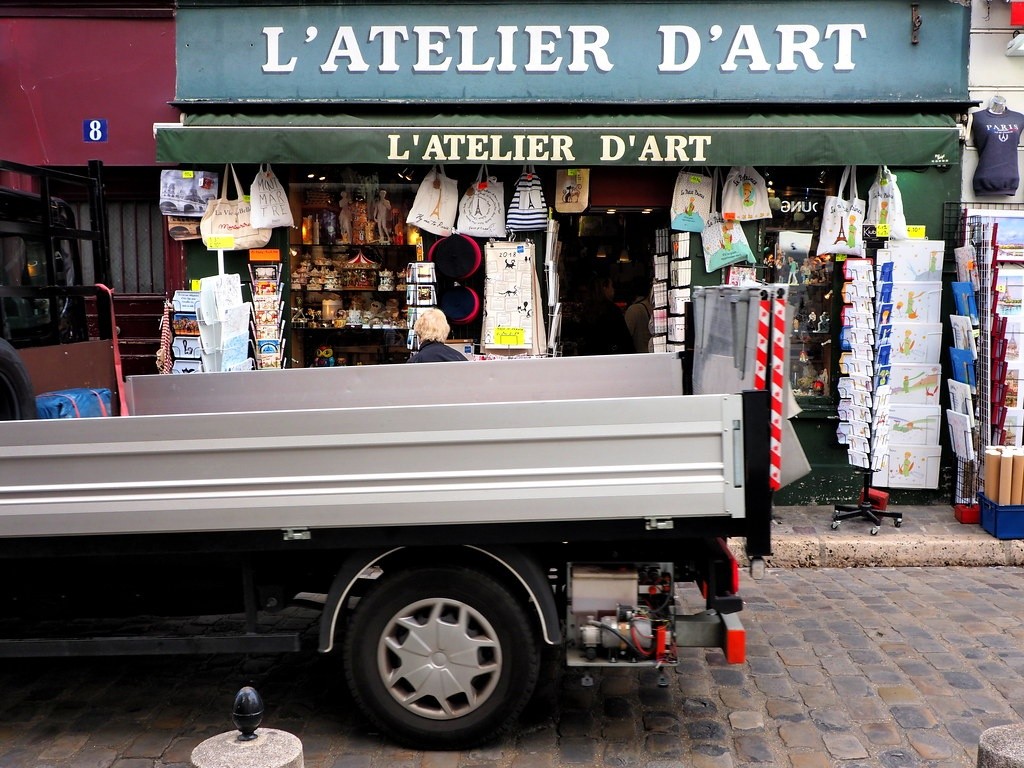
[818,168,831,183]
[397,167,415,180]
[597,215,606,257]
[305,166,325,181]
[619,220,630,263]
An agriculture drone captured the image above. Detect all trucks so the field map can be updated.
[1,332,747,748]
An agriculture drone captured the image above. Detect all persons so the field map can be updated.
[763,249,831,285]
[374,191,391,243]
[793,311,829,333]
[339,192,353,241]
[406,308,469,363]
[557,260,653,355]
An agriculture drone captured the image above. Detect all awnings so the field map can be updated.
[153,104,968,170]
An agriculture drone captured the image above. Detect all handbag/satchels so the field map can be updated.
[35,386,113,421]
[452,165,507,238]
[671,166,713,233]
[200,163,272,250]
[863,165,908,239]
[555,168,590,216]
[249,164,296,229]
[159,168,218,219]
[700,166,756,273]
[816,165,867,256]
[406,164,458,237]
[505,165,552,232]
[721,166,773,221]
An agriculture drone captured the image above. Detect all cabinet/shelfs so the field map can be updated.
[290,204,418,369]
[762,227,833,397]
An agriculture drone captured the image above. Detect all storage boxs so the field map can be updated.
[977,492,1024,540]
